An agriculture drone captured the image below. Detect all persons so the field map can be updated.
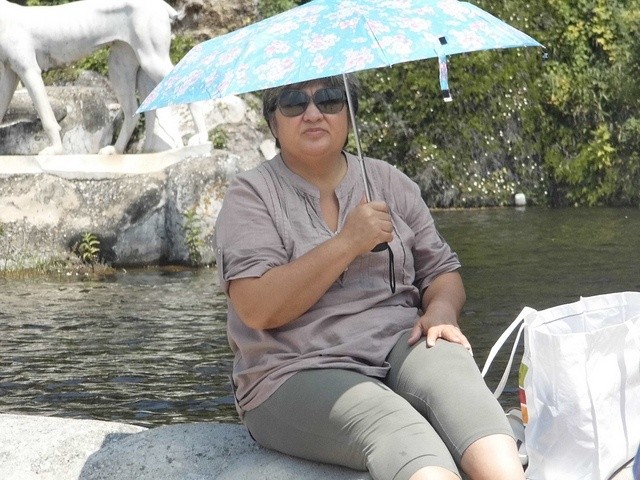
[213,74,526,480]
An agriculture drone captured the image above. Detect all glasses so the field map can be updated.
[271,88,348,117]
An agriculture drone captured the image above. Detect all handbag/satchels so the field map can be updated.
[480,291,640,480]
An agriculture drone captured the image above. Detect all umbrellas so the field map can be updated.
[132,0,548,294]
